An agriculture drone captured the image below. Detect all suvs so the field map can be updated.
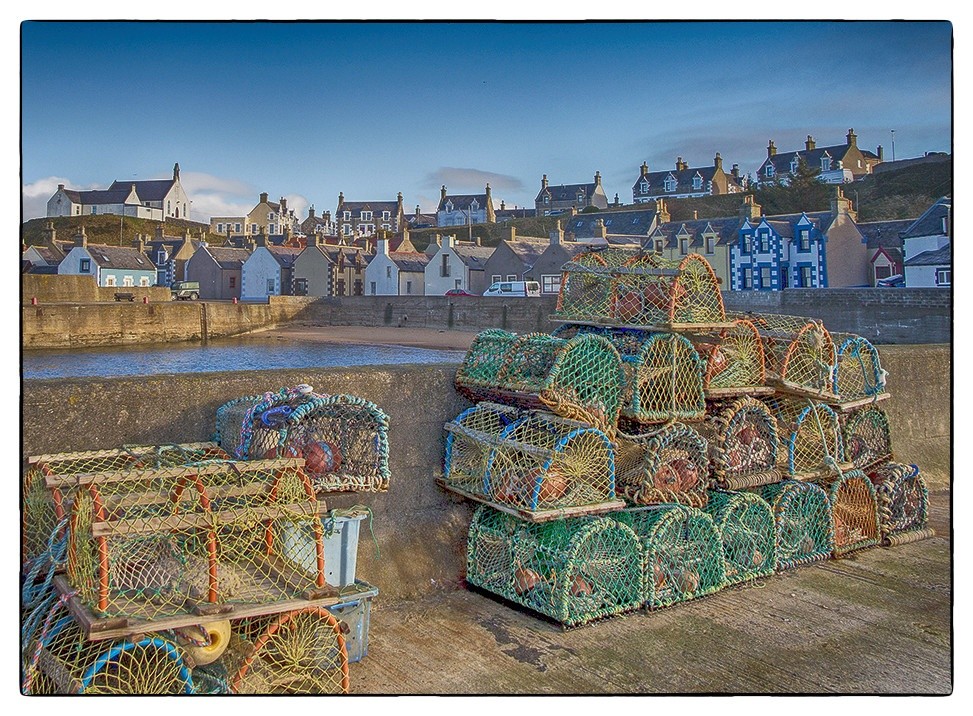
[170,280,200,301]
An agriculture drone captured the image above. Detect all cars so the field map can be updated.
[445,289,479,297]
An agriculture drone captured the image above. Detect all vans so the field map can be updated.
[482,281,541,298]
